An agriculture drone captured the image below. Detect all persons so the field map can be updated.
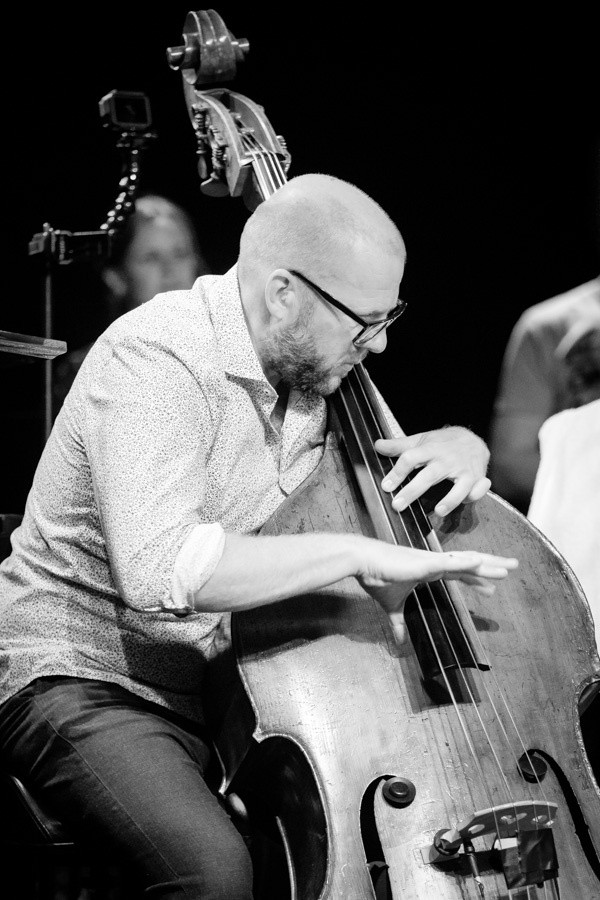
[105,195,600,658]
[1,174,521,900]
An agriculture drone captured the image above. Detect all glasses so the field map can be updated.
[289,270,407,348]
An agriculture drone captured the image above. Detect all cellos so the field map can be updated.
[162,6,600,900]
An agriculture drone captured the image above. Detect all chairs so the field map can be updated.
[0,515,259,900]
